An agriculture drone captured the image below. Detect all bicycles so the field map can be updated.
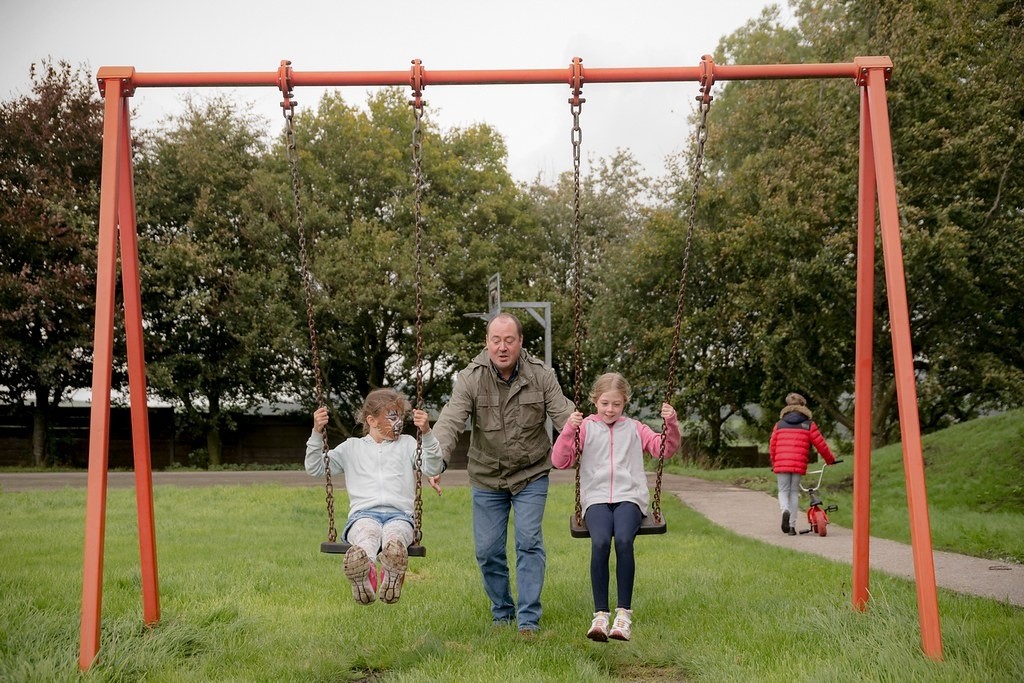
[770,458,844,537]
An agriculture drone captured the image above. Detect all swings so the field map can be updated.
[276,81,430,561]
[567,76,714,539]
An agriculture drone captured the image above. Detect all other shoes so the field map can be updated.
[519,629,535,637]
[788,527,796,536]
[781,510,791,534]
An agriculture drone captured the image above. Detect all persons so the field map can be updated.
[428,312,584,634]
[305,388,444,605]
[551,371,681,642]
[768,393,835,536]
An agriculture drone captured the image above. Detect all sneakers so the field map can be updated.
[344,544,377,605]
[609,608,633,641]
[379,539,408,604]
[587,610,610,642]
